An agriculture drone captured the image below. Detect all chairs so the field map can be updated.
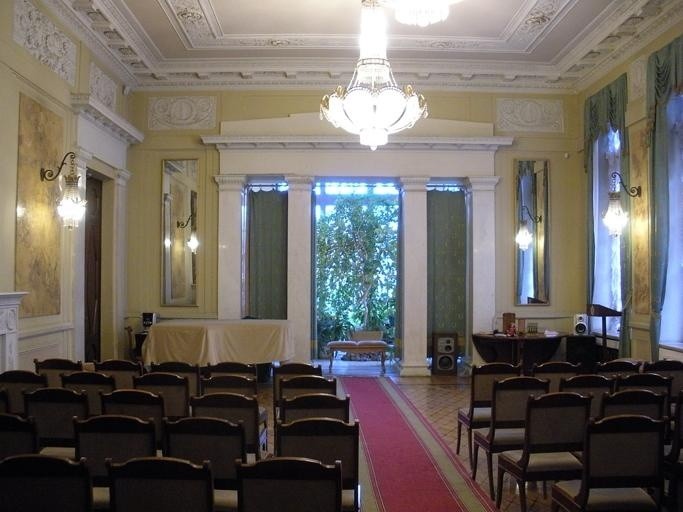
[271,362,322,416]
[100,389,166,422]
[71,416,158,487]
[3,455,91,511]
[33,359,85,390]
[274,418,361,511]
[151,360,198,396]
[106,459,215,511]
[276,374,338,408]
[201,378,258,402]
[189,393,269,462]
[199,363,265,406]
[233,455,340,511]
[22,388,95,461]
[123,326,142,363]
[134,375,193,421]
[2,413,37,461]
[457,358,683,512]
[59,371,117,413]
[274,392,351,433]
[92,359,145,390]
[2,371,48,420]
[160,417,247,491]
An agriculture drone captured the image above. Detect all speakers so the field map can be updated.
[574,313,589,335]
[432,331,458,375]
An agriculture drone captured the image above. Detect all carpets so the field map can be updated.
[330,375,501,512]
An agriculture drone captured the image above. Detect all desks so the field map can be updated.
[472,333,562,373]
[142,320,295,372]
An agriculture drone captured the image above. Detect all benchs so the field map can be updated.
[327,341,388,374]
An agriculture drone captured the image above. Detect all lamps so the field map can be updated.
[40,152,89,231]
[320,0,428,151]
[177,215,198,252]
[515,206,542,251]
[602,173,641,237]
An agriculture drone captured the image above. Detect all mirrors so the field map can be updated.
[514,159,549,306]
[160,158,198,307]
